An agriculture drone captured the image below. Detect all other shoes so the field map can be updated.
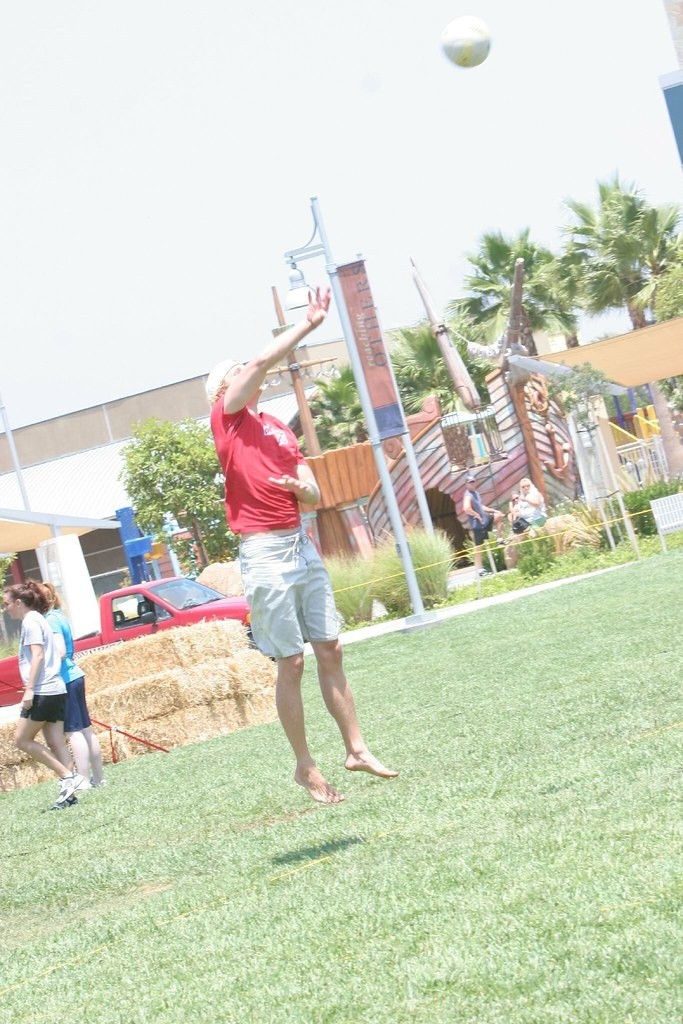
[90,777,106,787]
[480,570,492,577]
[78,781,91,792]
[498,539,510,544]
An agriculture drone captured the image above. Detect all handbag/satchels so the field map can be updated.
[512,518,529,534]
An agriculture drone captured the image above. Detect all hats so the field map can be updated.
[206,359,250,398]
[466,476,475,483]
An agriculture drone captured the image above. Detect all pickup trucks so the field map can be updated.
[0,576,274,708]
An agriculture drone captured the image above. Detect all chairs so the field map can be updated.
[113,600,157,629]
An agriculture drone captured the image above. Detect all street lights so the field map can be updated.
[284,197,427,625]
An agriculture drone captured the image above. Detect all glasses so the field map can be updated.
[512,496,518,502]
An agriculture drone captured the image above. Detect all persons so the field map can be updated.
[205,282,400,804]
[508,477,547,535]
[462,475,505,576]
[2,577,104,807]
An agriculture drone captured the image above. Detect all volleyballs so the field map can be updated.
[440,14,493,71]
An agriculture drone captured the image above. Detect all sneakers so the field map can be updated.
[55,771,85,804]
[51,797,78,812]
